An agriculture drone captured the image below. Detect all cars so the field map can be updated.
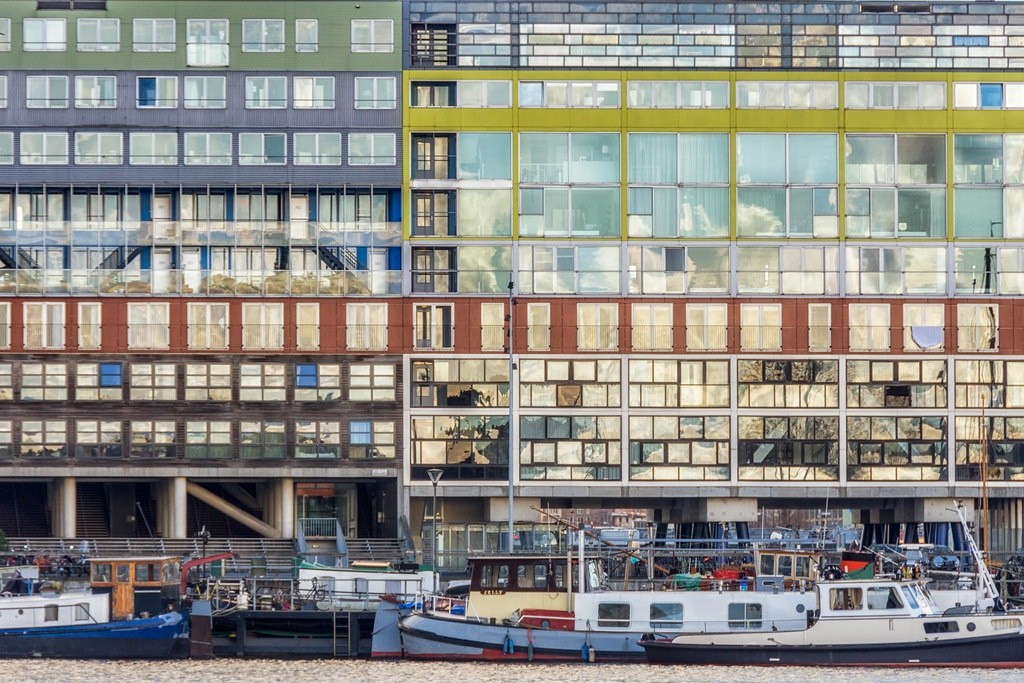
[869,543,905,560]
[925,544,962,567]
[903,549,929,568]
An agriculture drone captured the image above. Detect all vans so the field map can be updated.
[899,544,935,552]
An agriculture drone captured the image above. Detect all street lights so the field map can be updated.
[426,468,444,610]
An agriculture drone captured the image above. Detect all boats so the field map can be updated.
[0,502,1024,659]
[636,614,1023,664]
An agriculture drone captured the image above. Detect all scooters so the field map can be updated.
[58,554,74,578]
[74,554,91,576]
[5,546,28,566]
